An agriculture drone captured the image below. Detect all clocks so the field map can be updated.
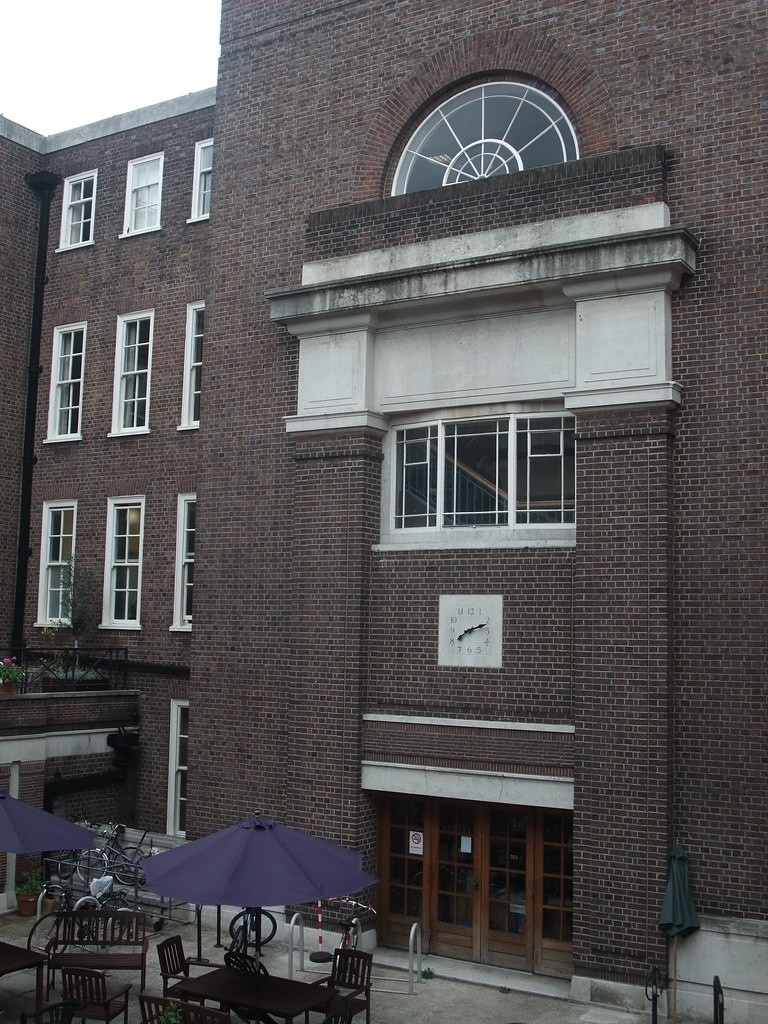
[438,594,503,669]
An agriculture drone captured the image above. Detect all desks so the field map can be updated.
[0,941,46,1024]
[175,966,339,1024]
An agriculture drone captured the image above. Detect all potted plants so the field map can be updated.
[15,867,44,916]
[41,552,109,692]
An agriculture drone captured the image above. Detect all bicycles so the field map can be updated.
[26,818,155,966]
[223,905,278,976]
[328,896,377,992]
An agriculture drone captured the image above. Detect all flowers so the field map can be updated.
[0,655,26,688]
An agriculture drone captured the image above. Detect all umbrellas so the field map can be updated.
[139,809,380,972]
[659,838,700,1024]
[0,794,97,854]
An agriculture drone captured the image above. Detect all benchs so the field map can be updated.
[45,910,149,1002]
[139,994,231,1024]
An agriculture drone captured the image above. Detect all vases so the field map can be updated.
[0,684,19,695]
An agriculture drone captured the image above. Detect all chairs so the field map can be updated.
[61,966,133,1024]
[304,948,374,1024]
[20,998,82,1024]
[224,952,277,1024]
[157,936,228,1009]
